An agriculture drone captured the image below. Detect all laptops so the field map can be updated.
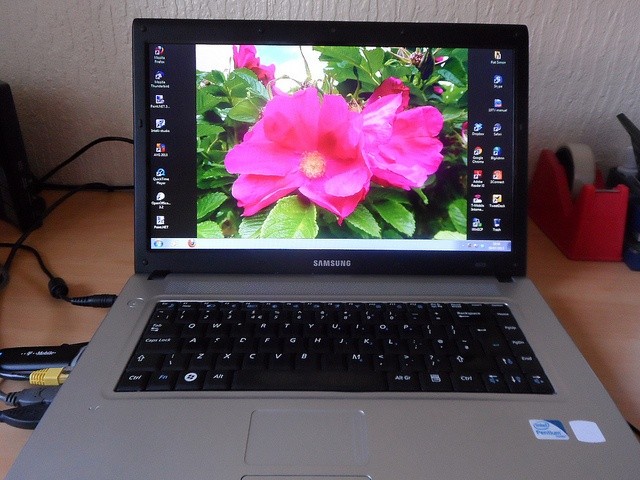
[0,17,639,480]
